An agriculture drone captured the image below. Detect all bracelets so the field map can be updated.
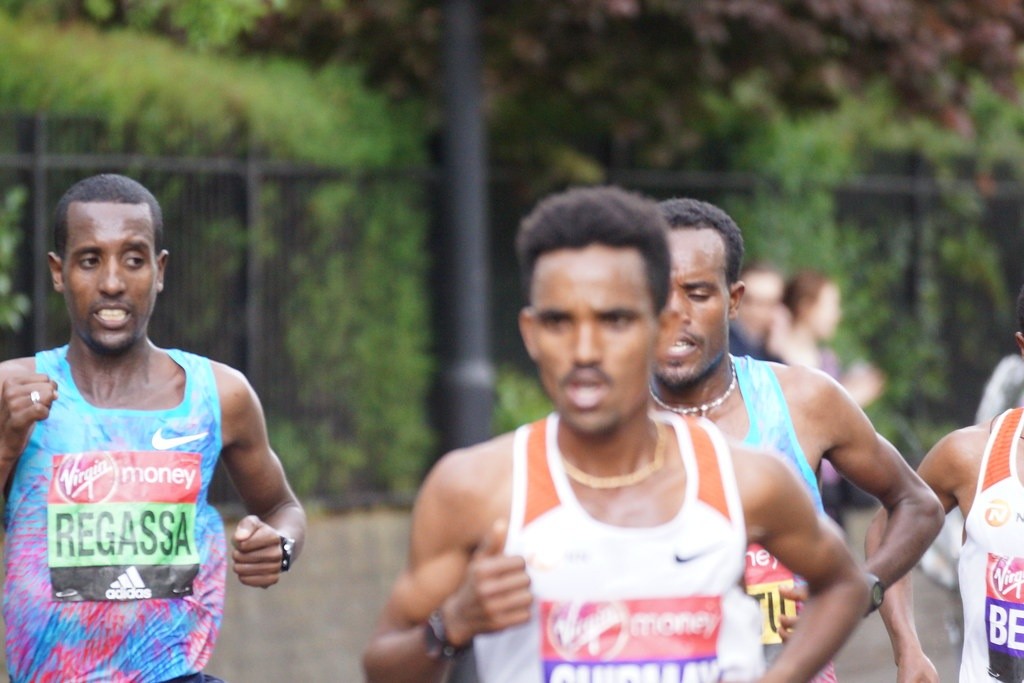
[422,615,461,663]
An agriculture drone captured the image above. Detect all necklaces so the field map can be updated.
[563,423,668,489]
[649,355,736,413]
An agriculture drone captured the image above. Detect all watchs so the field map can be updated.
[280,536,295,571]
[865,574,885,618]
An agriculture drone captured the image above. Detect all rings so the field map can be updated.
[31,391,39,403]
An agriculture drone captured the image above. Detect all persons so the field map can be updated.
[865,288,1024,683]
[362,184,945,683]
[0,173,306,683]
